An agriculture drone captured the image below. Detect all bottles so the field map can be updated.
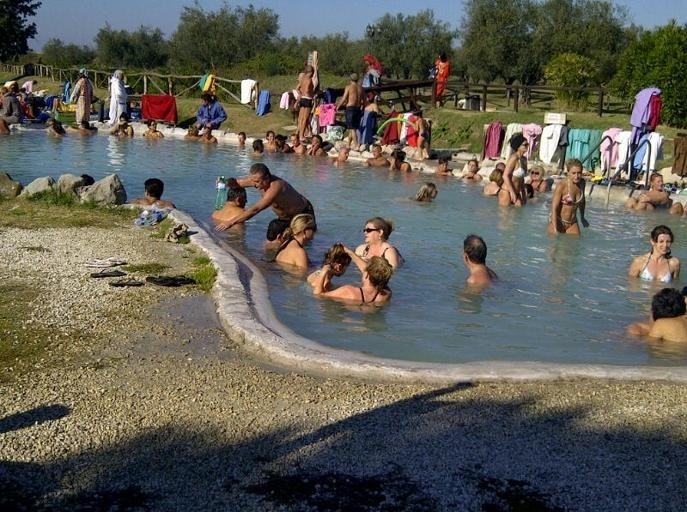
[135,200,172,227]
[213,175,229,209]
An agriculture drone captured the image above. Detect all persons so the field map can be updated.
[626,287,687,336]
[412,183,437,202]
[208,162,405,312]
[294,73,314,139]
[336,73,367,152]
[669,201,686,222]
[360,55,381,102]
[434,50,451,101]
[627,225,680,284]
[296,65,318,141]
[1,69,227,146]
[626,172,668,213]
[547,158,590,235]
[131,177,176,210]
[646,288,687,344]
[237,94,431,171]
[461,235,498,293]
[432,132,552,211]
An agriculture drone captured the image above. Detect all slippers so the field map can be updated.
[146,275,195,286]
[90,257,144,286]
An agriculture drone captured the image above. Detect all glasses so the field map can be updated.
[531,171,539,174]
[523,143,528,146]
[364,229,379,232]
